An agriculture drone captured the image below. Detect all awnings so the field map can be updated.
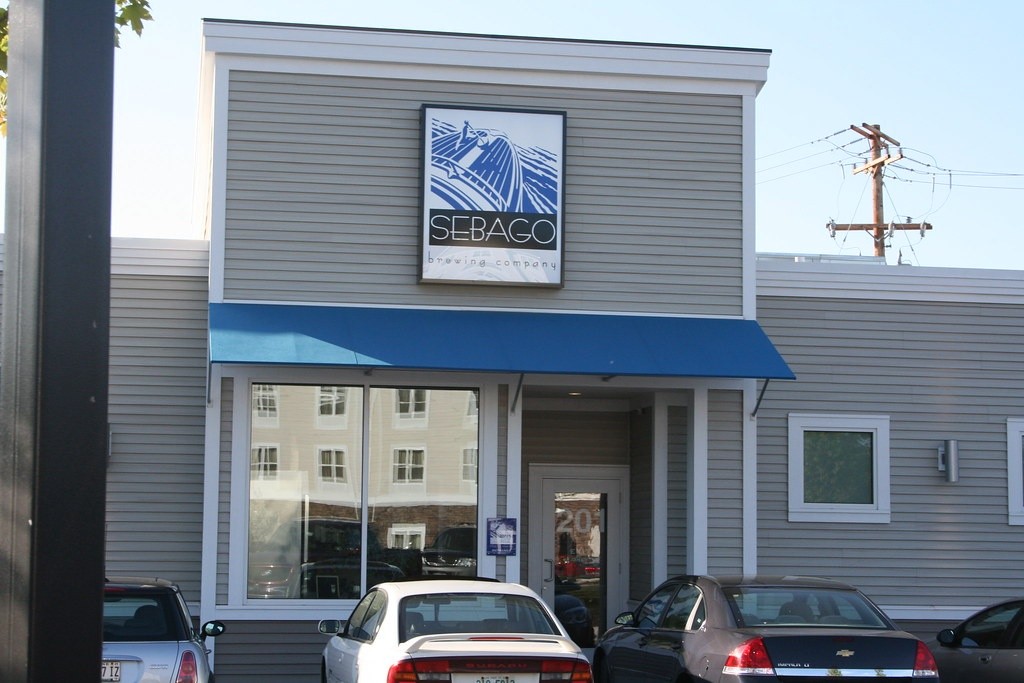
[207,302,796,417]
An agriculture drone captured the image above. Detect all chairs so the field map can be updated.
[126,604,170,642]
[740,600,852,625]
[405,610,424,634]
[483,619,508,632]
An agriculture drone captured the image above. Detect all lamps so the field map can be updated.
[938,440,960,483]
[568,386,585,398]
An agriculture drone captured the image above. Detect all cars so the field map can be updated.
[921,597,1024,683]
[100,574,227,683]
[248,513,477,599]
[554,550,601,632]
[590,571,942,683]
[316,578,595,683]
